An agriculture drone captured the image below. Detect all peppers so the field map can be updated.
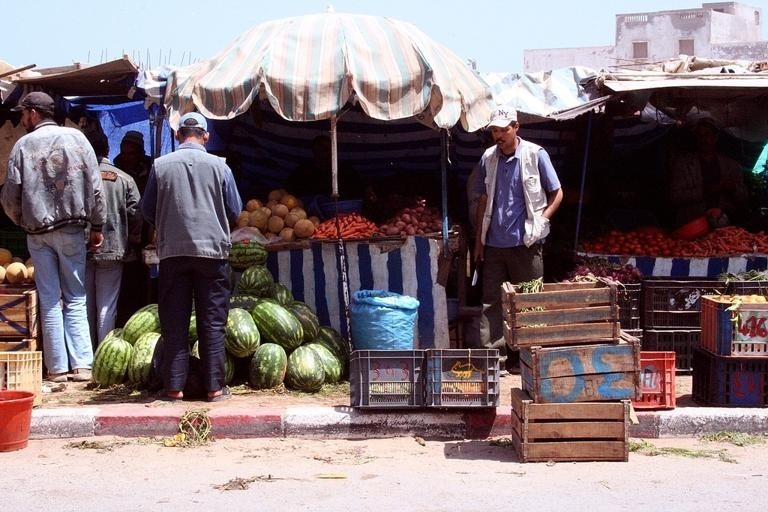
[682,226,768,257]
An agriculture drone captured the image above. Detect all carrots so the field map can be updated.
[312,212,379,239]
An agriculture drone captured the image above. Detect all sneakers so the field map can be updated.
[73,368,91,380]
[48,370,67,381]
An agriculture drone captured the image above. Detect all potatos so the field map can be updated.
[380,207,443,235]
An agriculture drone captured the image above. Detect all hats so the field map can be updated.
[10,92,55,114]
[487,107,517,128]
[180,112,207,131]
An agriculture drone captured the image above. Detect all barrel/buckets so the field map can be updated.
[0,390,37,453]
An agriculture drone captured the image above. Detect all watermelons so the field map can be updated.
[91,303,162,387]
[188,239,349,393]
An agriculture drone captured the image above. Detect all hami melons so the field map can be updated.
[0,248,34,283]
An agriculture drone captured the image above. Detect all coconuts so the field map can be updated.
[235,189,320,242]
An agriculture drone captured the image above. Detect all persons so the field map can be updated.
[667,117,749,226]
[112,129,155,200]
[140,110,243,403]
[1,91,108,383]
[81,128,142,353]
[312,137,358,199]
[466,105,564,374]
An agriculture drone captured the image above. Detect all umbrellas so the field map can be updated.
[136,3,496,195]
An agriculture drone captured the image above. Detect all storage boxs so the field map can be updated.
[0,281,43,409]
[502,277,622,349]
[620,277,767,372]
[525,332,642,403]
[428,345,503,411]
[347,344,426,413]
[692,348,767,407]
[508,386,630,465]
[631,350,678,411]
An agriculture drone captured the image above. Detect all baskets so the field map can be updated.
[619,276,768,409]
[0,351,42,407]
[350,349,500,409]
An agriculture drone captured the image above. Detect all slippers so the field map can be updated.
[208,387,231,402]
[154,389,182,401]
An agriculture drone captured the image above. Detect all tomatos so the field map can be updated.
[582,230,680,257]
